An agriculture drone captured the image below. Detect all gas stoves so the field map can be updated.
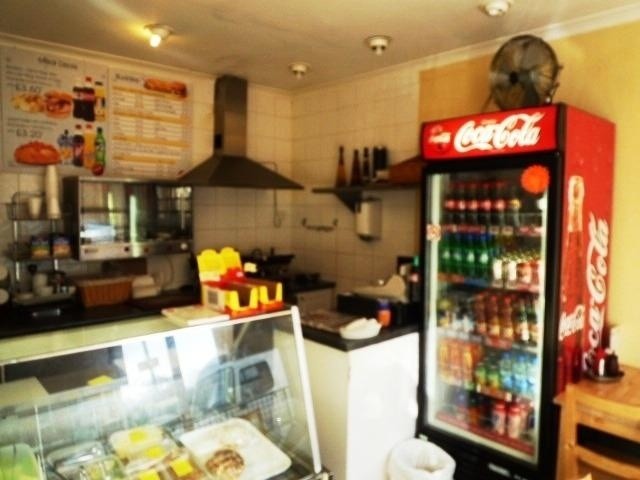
[240,271,334,304]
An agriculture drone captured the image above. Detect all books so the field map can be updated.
[159,302,230,327]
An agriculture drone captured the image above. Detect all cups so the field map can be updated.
[27,164,62,220]
[14,272,53,301]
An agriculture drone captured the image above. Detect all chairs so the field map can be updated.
[554,384,640,480]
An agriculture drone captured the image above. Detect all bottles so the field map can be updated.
[397,255,420,305]
[605,353,618,377]
[555,175,586,392]
[330,145,388,190]
[443,174,539,444]
[57,76,107,176]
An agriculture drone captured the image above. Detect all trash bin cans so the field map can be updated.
[388,438,456,480]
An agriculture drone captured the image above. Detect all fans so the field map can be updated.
[482,35,563,110]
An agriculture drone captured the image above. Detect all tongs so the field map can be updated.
[51,444,101,469]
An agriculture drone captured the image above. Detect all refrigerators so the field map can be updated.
[418,101,615,479]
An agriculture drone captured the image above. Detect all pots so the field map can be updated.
[238,246,295,272]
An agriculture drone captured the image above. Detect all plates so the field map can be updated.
[178,417,292,480]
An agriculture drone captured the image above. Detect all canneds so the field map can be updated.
[492,248,541,284]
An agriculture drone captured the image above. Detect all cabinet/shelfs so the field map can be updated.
[0,301,331,480]
[9,191,74,300]
[62,175,198,263]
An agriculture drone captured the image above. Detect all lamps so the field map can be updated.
[144,24,173,48]
[364,35,392,55]
[287,61,310,79]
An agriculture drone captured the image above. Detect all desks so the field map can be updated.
[550,361,640,431]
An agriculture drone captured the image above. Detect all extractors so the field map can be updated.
[169,73,305,189]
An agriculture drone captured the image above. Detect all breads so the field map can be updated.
[206,449,245,480]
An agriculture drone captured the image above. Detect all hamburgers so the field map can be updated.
[42,91,75,118]
